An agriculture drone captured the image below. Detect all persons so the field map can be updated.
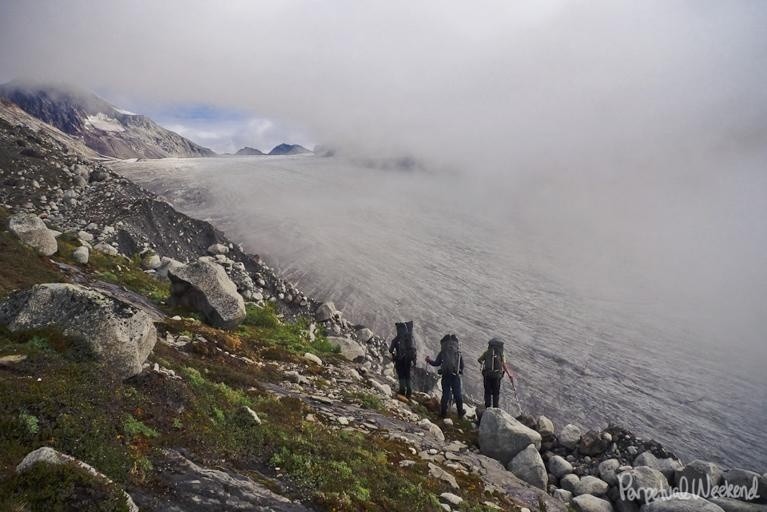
[426,335,466,418]
[478,340,512,407]
[389,321,416,398]
